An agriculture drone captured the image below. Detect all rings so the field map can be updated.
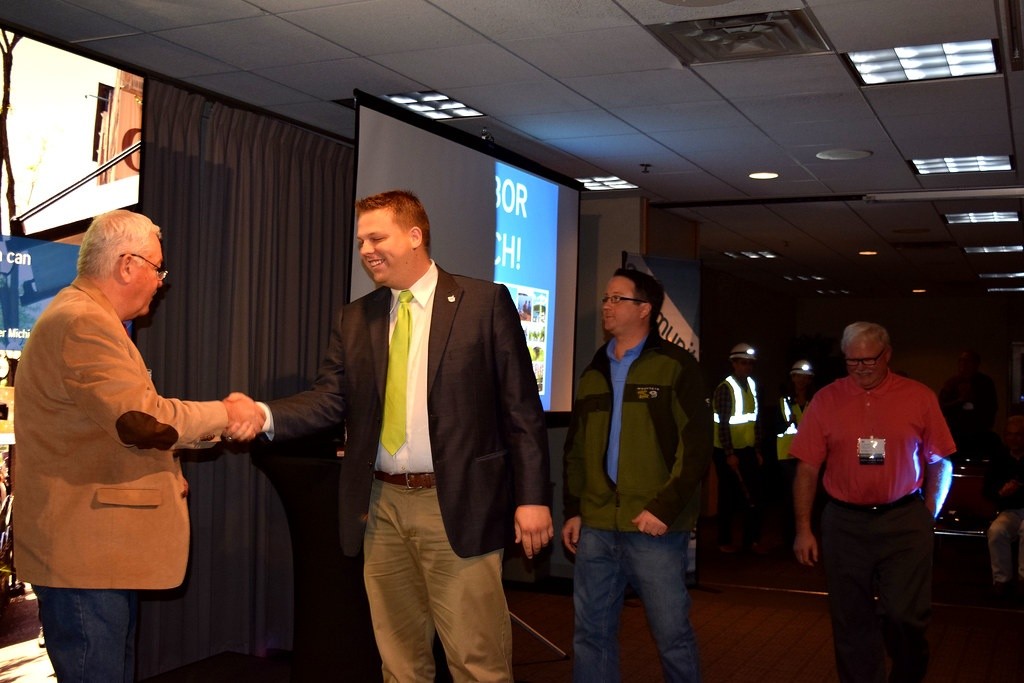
[228,437,232,440]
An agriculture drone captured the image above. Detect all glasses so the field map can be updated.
[119,252,170,280]
[600,294,649,305]
[842,349,885,365]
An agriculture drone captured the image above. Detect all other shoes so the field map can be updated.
[981,585,1008,606]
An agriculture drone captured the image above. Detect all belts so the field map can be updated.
[830,490,922,514]
[368,473,439,491]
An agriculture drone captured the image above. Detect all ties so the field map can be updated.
[380,290,414,457]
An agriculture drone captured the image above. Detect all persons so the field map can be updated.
[789,322,957,681]
[765,360,826,554]
[945,352,998,427]
[223,191,553,683]
[559,268,714,683]
[981,405,1023,606]
[710,343,763,553]
[11,210,256,683]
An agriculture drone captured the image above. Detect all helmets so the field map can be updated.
[790,360,814,375]
[729,342,757,360]
